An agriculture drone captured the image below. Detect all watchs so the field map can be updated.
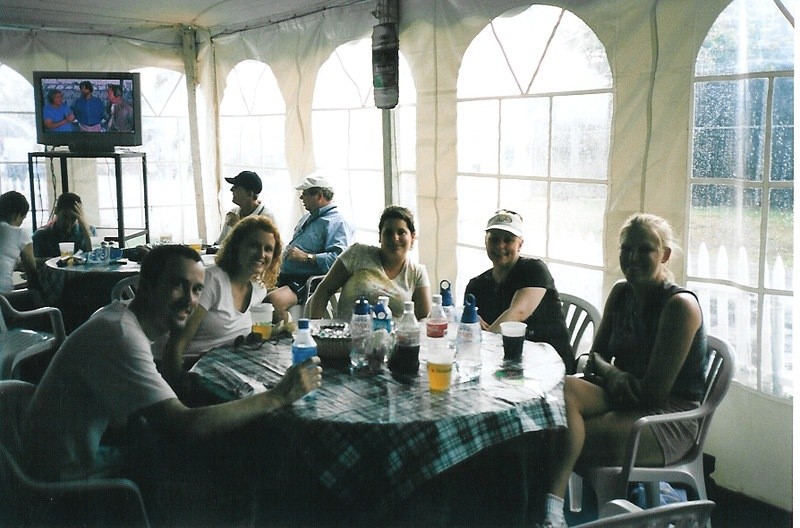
[307,253,313,264]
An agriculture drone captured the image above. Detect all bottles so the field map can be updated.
[349,296,373,368]
[454,294,483,380]
[372,295,393,333]
[426,294,448,353]
[438,279,456,350]
[291,318,318,398]
[395,301,421,374]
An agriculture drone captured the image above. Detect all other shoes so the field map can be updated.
[535,512,569,528]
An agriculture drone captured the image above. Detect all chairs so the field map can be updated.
[569,500,717,528]
[558,292,603,512]
[0,294,68,453]
[284,274,338,323]
[0,379,161,528]
[583,332,734,528]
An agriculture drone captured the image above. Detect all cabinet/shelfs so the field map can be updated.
[28,151,150,248]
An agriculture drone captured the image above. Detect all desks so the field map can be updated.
[35,252,219,334]
[186,324,571,528]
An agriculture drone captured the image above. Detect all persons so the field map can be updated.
[464,207,578,375]
[151,215,282,387]
[213,171,276,245]
[0,190,97,316]
[532,214,708,528]
[307,205,429,321]
[264,173,355,324]
[17,244,323,528]
[43,81,134,132]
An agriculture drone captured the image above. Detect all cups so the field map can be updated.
[89,236,105,250]
[288,322,321,340]
[500,322,527,358]
[59,242,74,265]
[248,303,275,340]
[160,235,172,242]
[365,336,393,373]
[188,239,203,253]
[425,347,454,391]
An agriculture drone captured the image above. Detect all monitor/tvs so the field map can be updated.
[33,71,141,153]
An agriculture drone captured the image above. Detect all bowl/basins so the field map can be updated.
[312,323,351,361]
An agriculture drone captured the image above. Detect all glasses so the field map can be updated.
[234,332,281,350]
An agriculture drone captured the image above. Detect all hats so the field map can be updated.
[295,173,331,191]
[484,212,523,239]
[224,171,262,193]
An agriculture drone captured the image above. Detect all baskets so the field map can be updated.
[311,324,351,361]
